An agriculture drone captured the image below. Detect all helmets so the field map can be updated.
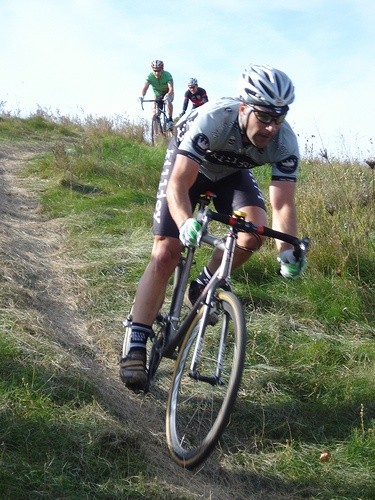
[151,60,164,69]
[186,77,197,86]
[241,64,295,107]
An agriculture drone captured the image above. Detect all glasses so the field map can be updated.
[188,86,196,89]
[153,69,162,72]
[252,109,288,126]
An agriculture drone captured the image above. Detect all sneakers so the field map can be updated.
[183,280,220,326]
[119,345,148,392]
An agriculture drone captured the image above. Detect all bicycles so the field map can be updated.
[139,96,175,146]
[141,192,312,469]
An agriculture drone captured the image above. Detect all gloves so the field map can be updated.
[138,95,144,104]
[276,248,308,281]
[180,112,185,117]
[163,94,170,103]
[178,217,203,248]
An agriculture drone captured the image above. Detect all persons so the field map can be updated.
[174,78,208,124]
[139,59,174,131]
[118,63,304,391]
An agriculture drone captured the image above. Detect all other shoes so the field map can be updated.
[168,121,174,130]
